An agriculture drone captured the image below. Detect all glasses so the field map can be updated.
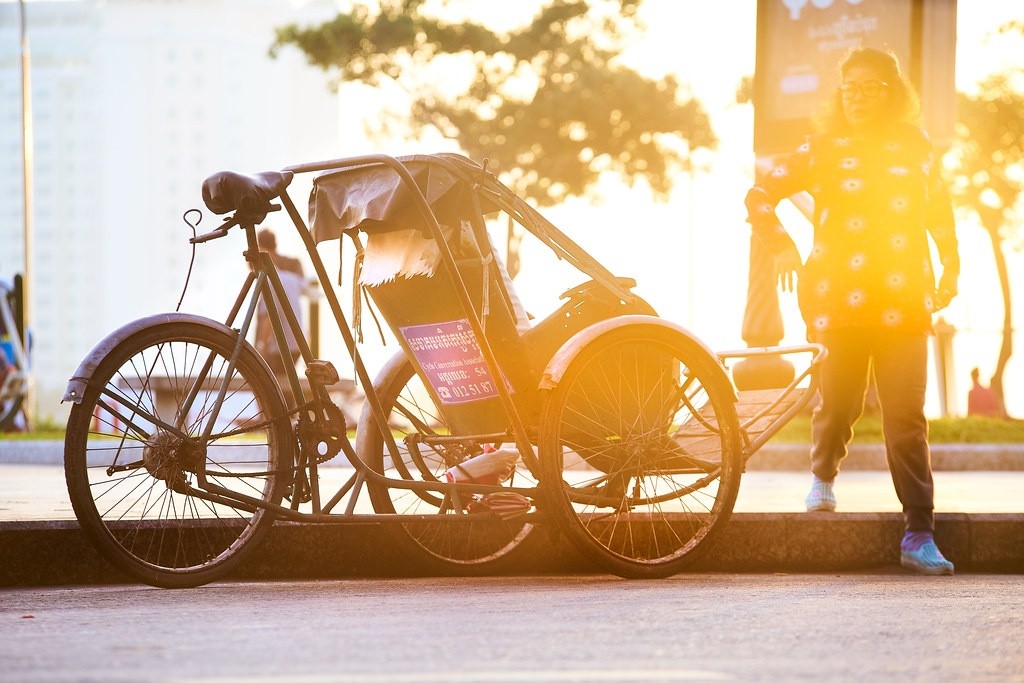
[837,79,890,100]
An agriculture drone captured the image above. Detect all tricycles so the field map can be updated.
[61,149,829,588]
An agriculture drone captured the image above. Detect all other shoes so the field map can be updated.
[806,476,836,512]
[899,531,954,575]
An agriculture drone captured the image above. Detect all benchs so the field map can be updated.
[118,376,357,434]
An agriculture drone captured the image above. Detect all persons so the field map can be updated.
[254,229,300,417]
[746,48,961,576]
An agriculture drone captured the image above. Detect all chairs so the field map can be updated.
[309,153,637,438]
